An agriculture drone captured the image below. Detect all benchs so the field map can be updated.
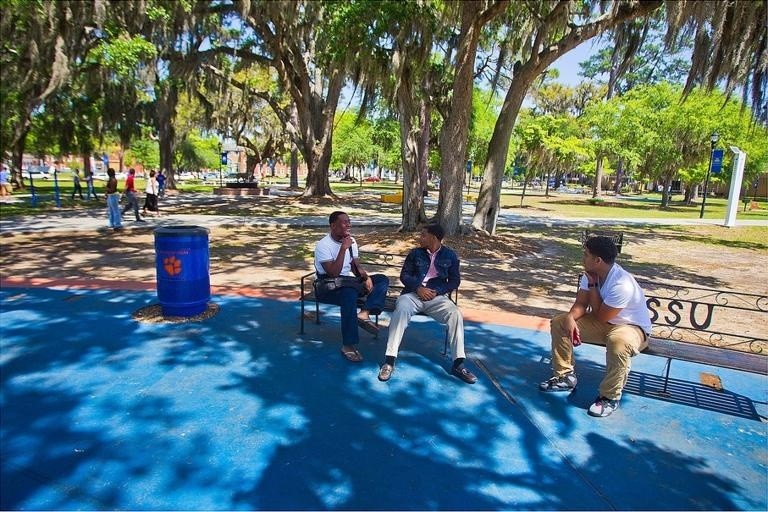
[299,251,460,355]
[577,275,768,393]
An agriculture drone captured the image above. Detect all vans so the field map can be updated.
[20,171,51,181]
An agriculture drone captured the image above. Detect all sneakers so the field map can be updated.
[539,373,577,392]
[588,396,619,417]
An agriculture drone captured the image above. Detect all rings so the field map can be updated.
[426,292,429,295]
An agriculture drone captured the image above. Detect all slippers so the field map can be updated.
[340,350,362,362]
[356,317,379,335]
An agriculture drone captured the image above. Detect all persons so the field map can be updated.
[378,222,478,384]
[122,168,146,222]
[314,211,389,363]
[72,170,84,200]
[83,171,100,201]
[0,168,13,196]
[141,170,161,217]
[155,171,167,199]
[539,237,652,418]
[103,168,123,229]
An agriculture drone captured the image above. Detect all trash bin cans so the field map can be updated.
[154,226,211,317]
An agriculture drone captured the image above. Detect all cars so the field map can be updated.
[341,175,358,182]
[654,183,673,194]
[362,175,382,183]
[92,172,256,181]
[56,167,72,173]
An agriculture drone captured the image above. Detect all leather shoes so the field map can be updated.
[451,365,476,384]
[378,362,395,381]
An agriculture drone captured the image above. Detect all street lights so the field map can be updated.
[467,152,475,195]
[101,151,109,172]
[217,142,223,186]
[700,132,719,219]
